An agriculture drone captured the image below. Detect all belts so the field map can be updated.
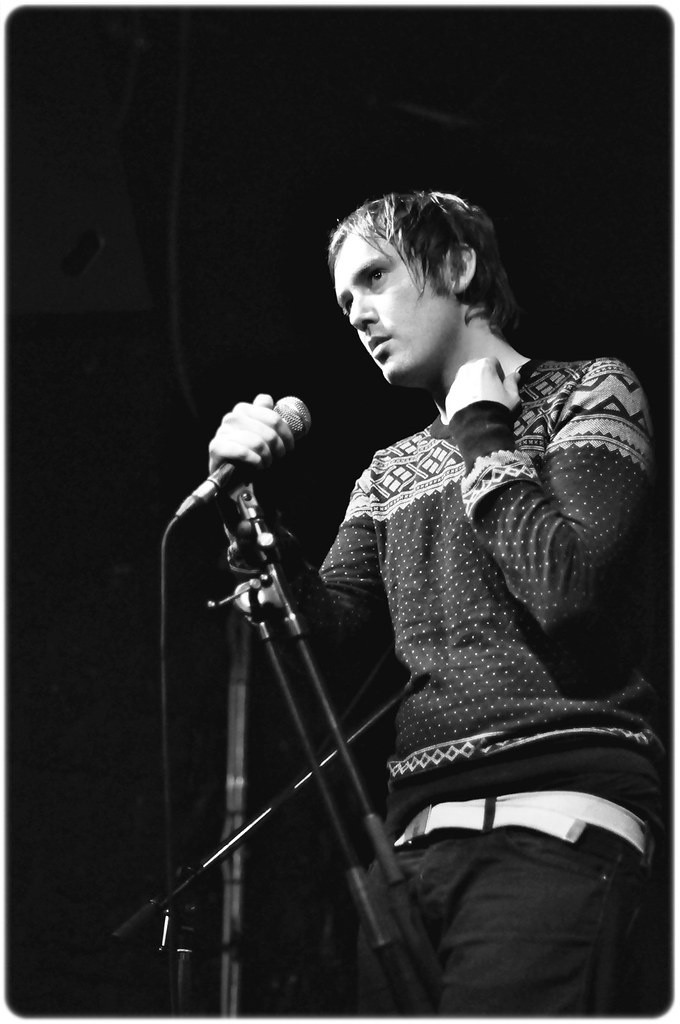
[393,790,647,853]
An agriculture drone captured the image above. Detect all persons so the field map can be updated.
[209,192,673,1018]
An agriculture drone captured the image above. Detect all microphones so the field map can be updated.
[156,670,434,909]
[174,396,312,521]
